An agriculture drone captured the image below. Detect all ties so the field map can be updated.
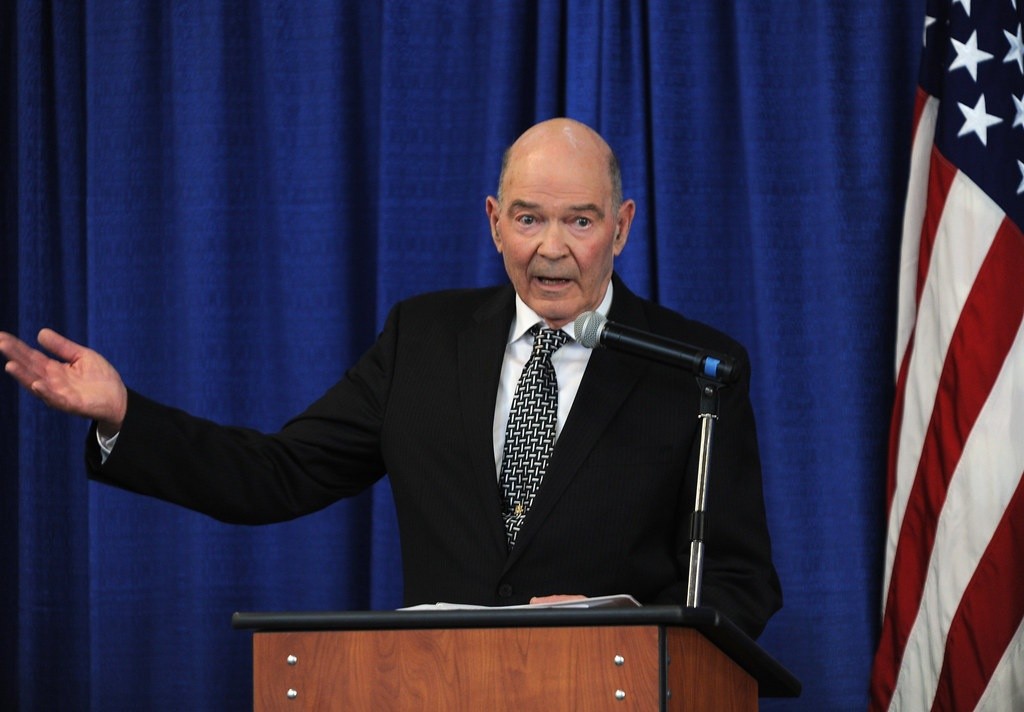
[497,325,576,549]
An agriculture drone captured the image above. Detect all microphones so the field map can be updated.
[572,310,739,383]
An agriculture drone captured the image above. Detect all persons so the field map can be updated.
[0,118,784,642]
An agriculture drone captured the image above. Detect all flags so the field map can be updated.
[869,0,1024,712]
